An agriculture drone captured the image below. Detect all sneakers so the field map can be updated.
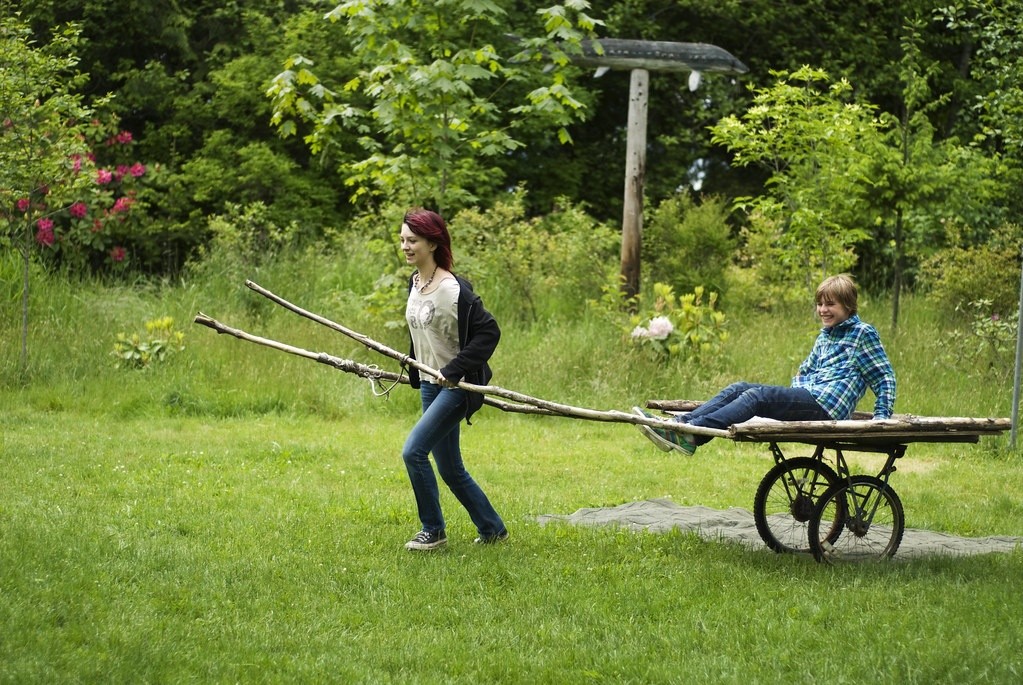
[404,529,448,550]
[473,528,509,543]
[631,406,697,456]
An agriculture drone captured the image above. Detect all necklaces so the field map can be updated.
[414,266,438,292]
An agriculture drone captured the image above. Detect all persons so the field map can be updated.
[632,273,897,457]
[401,209,508,550]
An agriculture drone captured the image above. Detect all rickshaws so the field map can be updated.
[192,277,1013,565]
